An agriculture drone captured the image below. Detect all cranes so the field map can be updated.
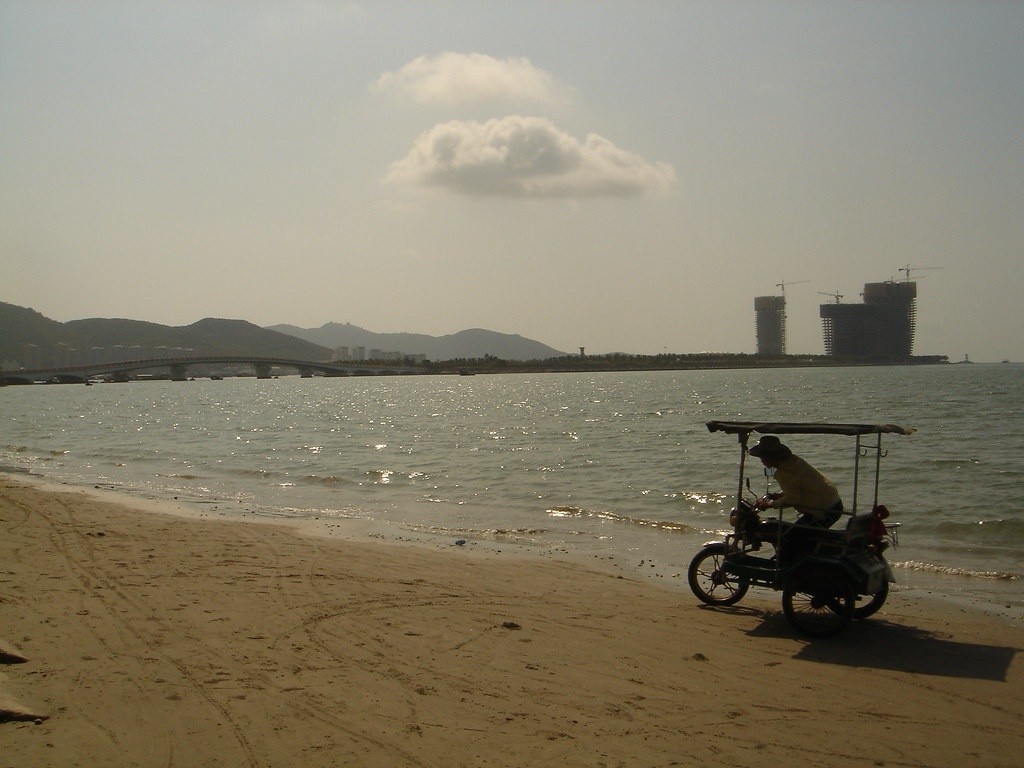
[896,265,944,281]
[775,278,810,297]
[817,289,844,303]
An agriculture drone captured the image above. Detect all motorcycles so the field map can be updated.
[685,419,918,641]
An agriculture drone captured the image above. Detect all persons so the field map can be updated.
[748,436,843,562]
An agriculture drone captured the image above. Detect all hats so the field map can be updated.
[748,436,791,456]
